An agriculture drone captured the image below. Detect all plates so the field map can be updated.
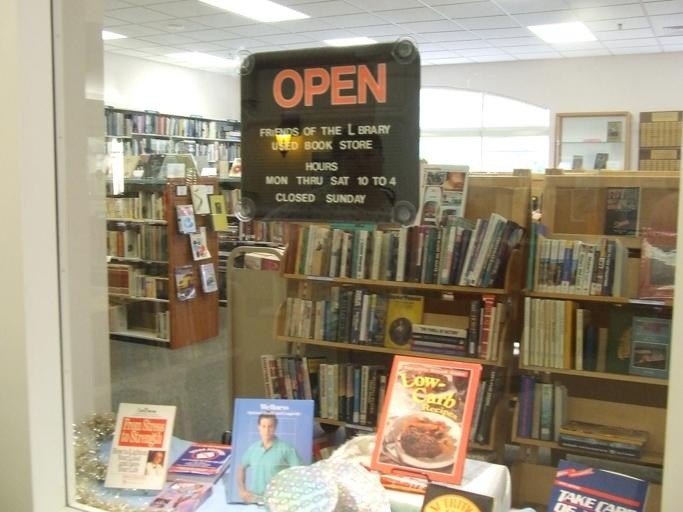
[385,409,461,469]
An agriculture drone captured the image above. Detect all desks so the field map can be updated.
[72,432,516,512]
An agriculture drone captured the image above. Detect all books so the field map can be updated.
[607,120,682,171]
[105,355,651,512]
[103,109,246,342]
[262,153,676,457]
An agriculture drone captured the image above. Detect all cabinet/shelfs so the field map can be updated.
[231,164,680,486]
[105,103,242,177]
[107,172,220,352]
[206,174,284,306]
